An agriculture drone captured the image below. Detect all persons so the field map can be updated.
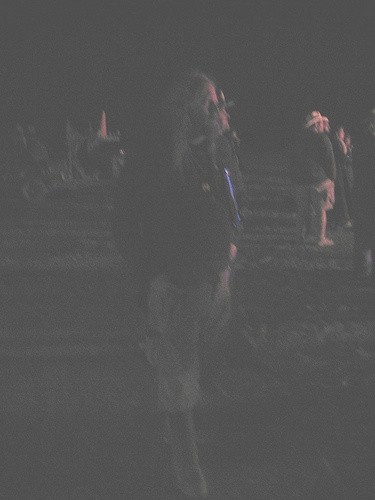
[1,87,244,321]
[111,67,242,499]
[291,108,375,290]
